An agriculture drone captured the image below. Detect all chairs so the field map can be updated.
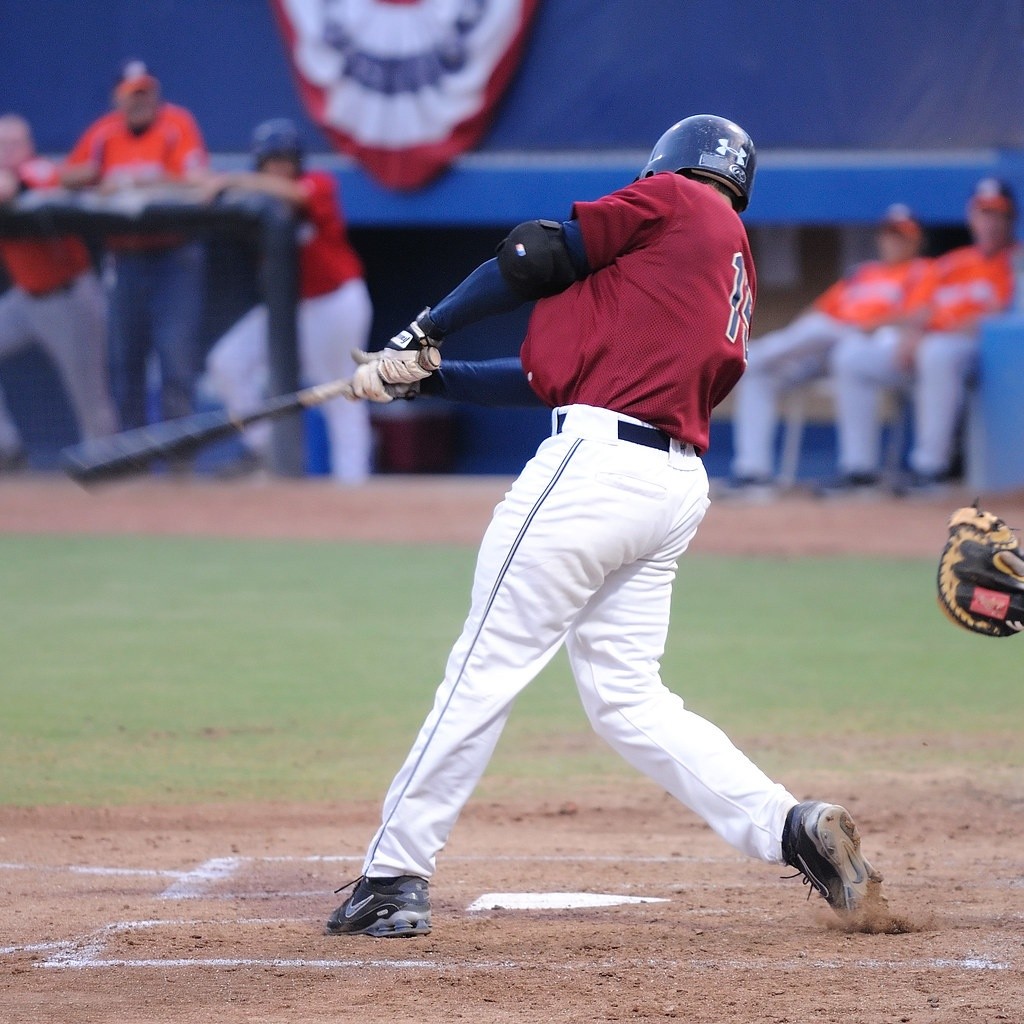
[775,364,918,486]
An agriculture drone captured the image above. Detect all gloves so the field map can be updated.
[340,362,421,402]
[348,322,441,383]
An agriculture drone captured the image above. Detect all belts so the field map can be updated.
[557,414,699,455]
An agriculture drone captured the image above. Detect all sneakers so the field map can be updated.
[323,874,433,938]
[779,800,888,923]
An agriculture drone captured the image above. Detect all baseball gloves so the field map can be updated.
[938,502,1024,639]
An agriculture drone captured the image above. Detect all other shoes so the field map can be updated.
[818,472,880,499]
[720,477,769,489]
[891,472,947,498]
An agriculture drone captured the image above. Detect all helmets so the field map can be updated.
[631,113,756,213]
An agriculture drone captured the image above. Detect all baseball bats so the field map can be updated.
[55,344,443,496]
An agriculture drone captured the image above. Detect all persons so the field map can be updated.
[323,116,889,939]
[0,62,1024,499]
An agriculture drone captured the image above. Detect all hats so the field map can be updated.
[113,60,160,100]
[873,203,920,242]
[966,179,1015,215]
[250,119,305,169]
